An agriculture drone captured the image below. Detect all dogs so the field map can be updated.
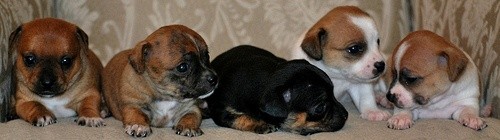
[100,24,220,139]
[292,6,395,122]
[6,18,111,128]
[386,30,492,131]
[204,45,349,136]
